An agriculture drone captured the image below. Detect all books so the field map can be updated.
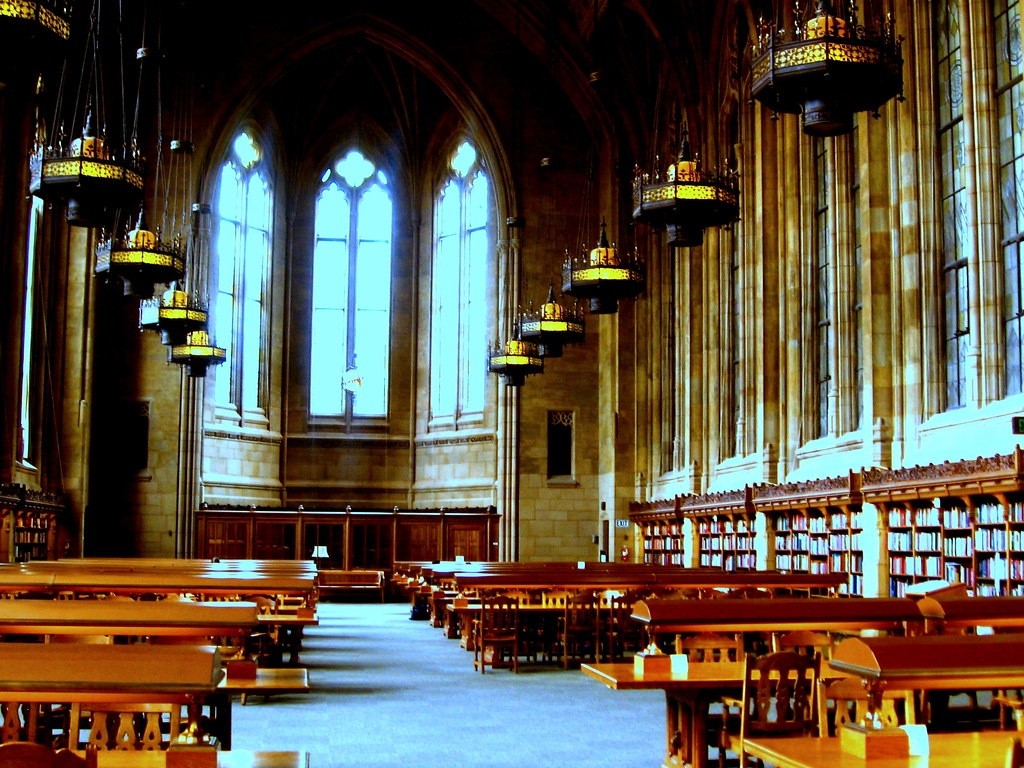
[13,516,51,563]
[643,501,1023,599]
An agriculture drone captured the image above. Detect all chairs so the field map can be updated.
[468,595,521,673]
[547,594,600,671]
[813,678,915,739]
[0,700,38,744]
[69,696,178,752]
[236,594,280,651]
[602,595,646,662]
[771,632,833,739]
[716,651,820,768]
[671,629,743,768]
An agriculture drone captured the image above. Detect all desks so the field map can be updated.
[1,559,1023,768]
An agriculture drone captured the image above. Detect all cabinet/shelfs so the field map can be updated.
[0,483,75,559]
[630,473,1024,595]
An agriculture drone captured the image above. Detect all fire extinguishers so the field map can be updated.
[621,544,629,562]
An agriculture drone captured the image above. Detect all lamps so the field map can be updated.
[515,155,587,359]
[630,1,747,248]
[743,0,903,142]
[562,72,648,314]
[28,0,224,375]
[486,216,546,384]
